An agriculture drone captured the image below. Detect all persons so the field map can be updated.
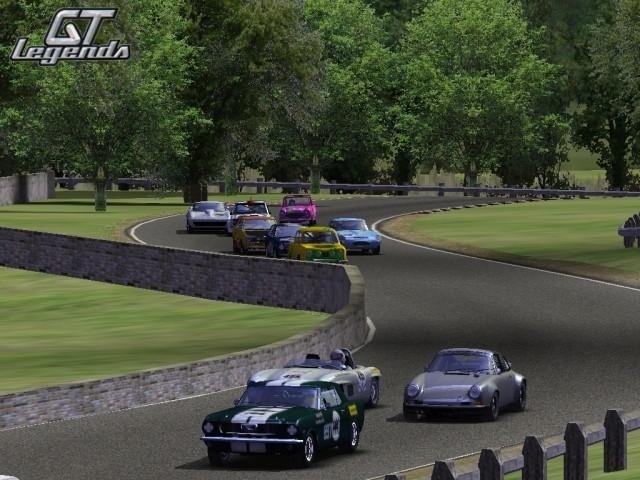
[303,390,322,408]
[330,349,353,370]
